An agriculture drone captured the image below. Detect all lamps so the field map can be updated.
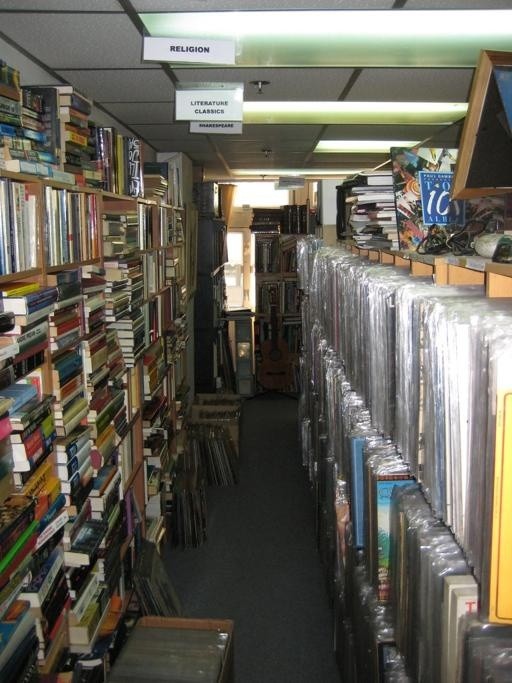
[241,101,469,126]
[137,9,512,68]
[314,140,420,154]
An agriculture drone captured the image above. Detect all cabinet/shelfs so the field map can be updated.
[1,169,193,676]
[194,216,236,389]
[252,230,311,397]
[340,229,512,295]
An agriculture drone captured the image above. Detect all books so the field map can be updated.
[145,297,160,343]
[0,178,102,276]
[91,126,144,194]
[142,396,173,468]
[256,234,294,355]
[143,344,171,401]
[145,161,168,203]
[295,235,511,682]
[1,283,127,682]
[335,172,511,258]
[249,205,311,232]
[104,212,145,368]
[0,64,98,187]
[139,207,187,247]
[148,249,181,294]
[162,294,191,362]
